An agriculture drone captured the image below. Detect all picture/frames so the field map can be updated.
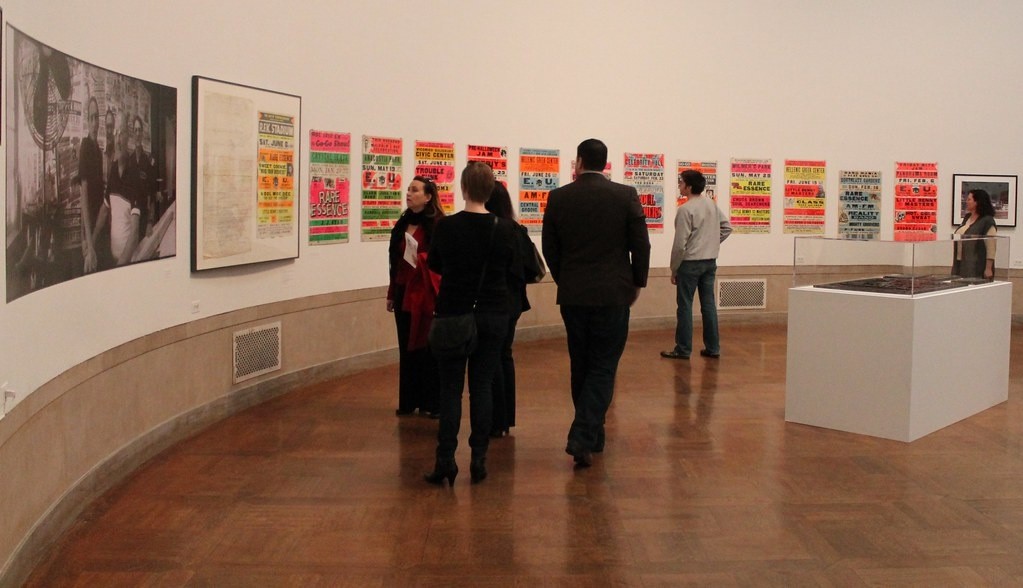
[952,174,1018,228]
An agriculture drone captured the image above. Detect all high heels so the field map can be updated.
[424,464,458,487]
[470,463,487,484]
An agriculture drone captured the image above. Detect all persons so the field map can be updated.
[541,138,652,468]
[483,181,532,440]
[659,169,733,360]
[79,95,106,271]
[81,111,168,266]
[951,188,997,282]
[386,175,448,420]
[423,160,517,487]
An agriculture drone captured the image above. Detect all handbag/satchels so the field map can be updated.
[527,243,545,283]
[429,316,477,347]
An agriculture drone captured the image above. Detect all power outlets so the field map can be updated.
[0,383,8,420]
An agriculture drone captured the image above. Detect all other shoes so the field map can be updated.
[660,350,689,359]
[396,405,415,416]
[591,440,605,452]
[565,440,592,466]
[700,349,720,358]
